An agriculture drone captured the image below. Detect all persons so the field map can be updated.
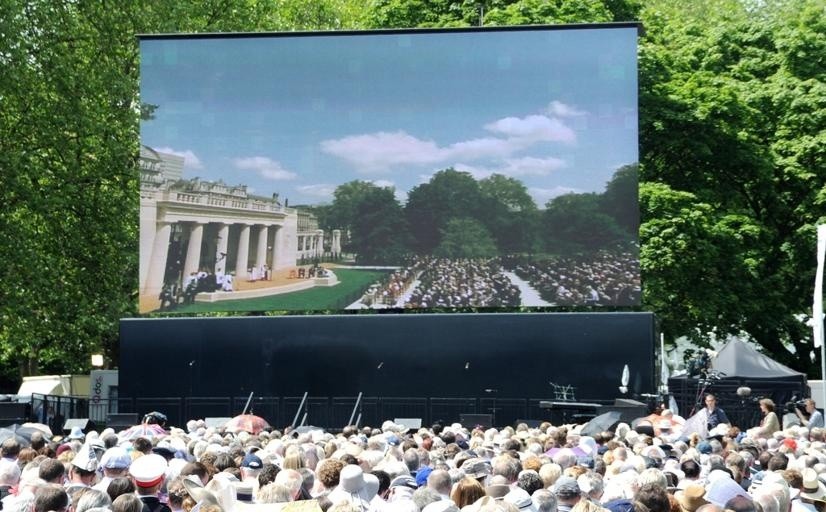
[359,239,641,307]
[1,388,825,512]
[289,267,328,279]
[183,263,274,293]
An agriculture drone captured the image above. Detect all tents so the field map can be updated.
[667,334,811,432]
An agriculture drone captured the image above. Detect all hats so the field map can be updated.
[1,408,826,512]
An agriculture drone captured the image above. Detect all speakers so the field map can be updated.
[106,413,139,433]
[0,403,31,427]
[597,405,647,423]
[459,413,492,428]
